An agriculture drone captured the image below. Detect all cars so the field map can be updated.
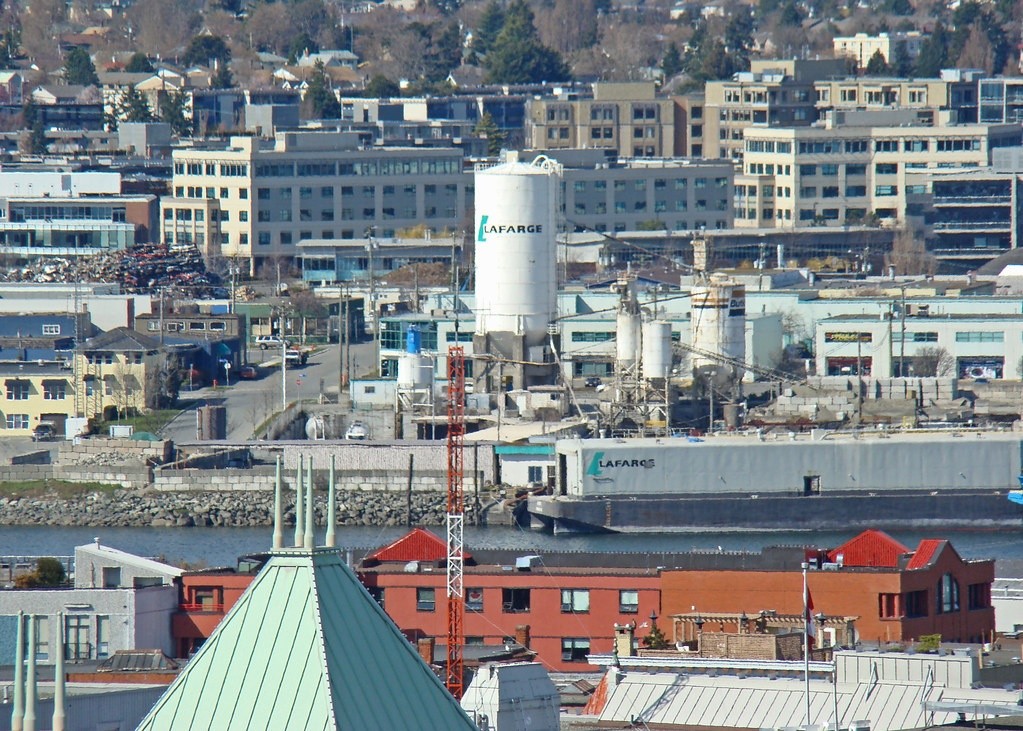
[286,350,309,365]
[32,425,57,441]
[254,336,293,349]
[241,366,258,379]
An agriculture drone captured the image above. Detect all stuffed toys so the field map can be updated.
[802,583,816,651]
